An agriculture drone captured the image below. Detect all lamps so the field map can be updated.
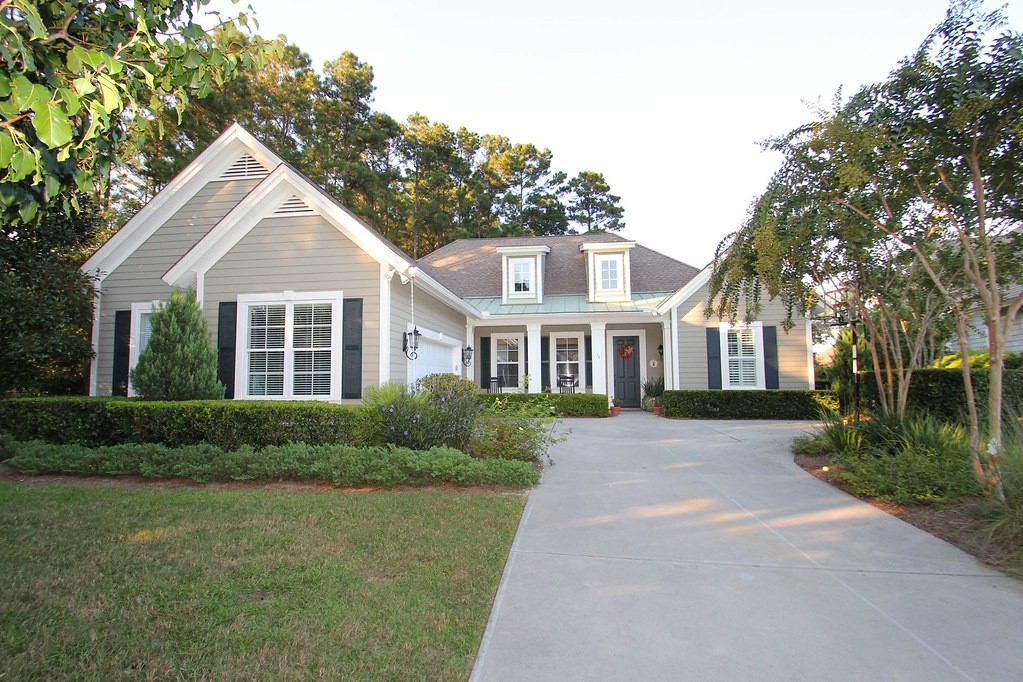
[398,272,410,285]
[462,345,473,367]
[384,269,396,282]
[402,326,422,360]
[656,344,663,356]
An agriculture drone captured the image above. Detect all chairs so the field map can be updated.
[487,377,502,393]
[559,375,575,394]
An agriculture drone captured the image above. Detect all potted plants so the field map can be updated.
[610,399,624,415]
[654,397,662,415]
